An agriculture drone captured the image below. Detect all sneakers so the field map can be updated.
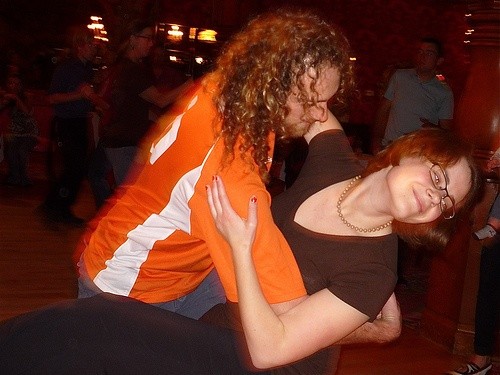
[447,360,493,375]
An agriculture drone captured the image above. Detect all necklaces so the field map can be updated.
[337,174,393,233]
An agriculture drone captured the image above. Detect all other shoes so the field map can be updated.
[41,207,85,227]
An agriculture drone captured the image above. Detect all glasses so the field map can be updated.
[424,154,456,219]
[134,36,153,41]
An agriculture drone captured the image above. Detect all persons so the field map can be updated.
[0,21,193,234]
[446,148,500,375]
[375,37,455,151]
[76,12,400,343]
[0,109,476,375]
